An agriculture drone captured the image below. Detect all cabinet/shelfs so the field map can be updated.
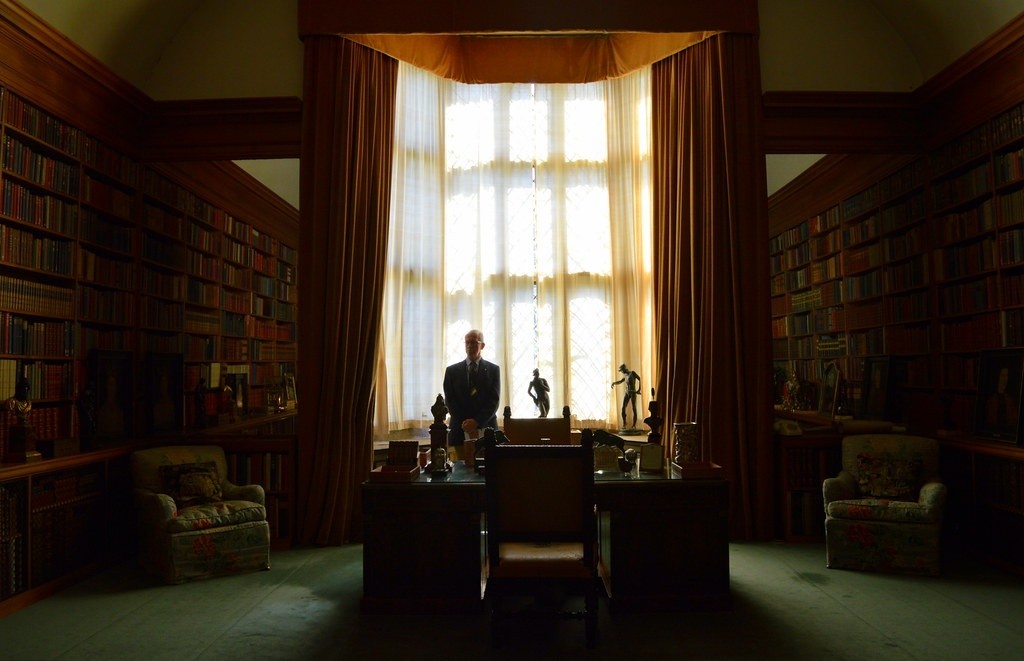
[0,57,300,619]
[764,77,1024,579]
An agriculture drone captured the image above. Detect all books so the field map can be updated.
[768,102,1023,447]
[0,85,298,597]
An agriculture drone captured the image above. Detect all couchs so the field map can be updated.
[132,445,271,588]
[823,433,949,577]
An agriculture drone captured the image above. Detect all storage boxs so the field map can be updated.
[369,441,420,483]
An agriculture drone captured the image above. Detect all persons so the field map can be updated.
[528,368,551,418]
[611,364,641,428]
[443,330,501,446]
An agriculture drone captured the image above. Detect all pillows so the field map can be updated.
[157,459,222,511]
[856,449,921,502]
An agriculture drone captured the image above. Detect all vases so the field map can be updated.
[674,422,700,464]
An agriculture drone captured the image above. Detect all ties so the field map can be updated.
[469,362,477,384]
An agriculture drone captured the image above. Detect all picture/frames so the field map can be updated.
[816,361,842,422]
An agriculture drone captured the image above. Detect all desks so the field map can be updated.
[773,408,893,546]
[360,458,737,615]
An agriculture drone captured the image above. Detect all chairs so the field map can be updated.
[483,425,601,653]
[501,406,572,445]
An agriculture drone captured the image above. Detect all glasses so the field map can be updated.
[465,340,482,345]
